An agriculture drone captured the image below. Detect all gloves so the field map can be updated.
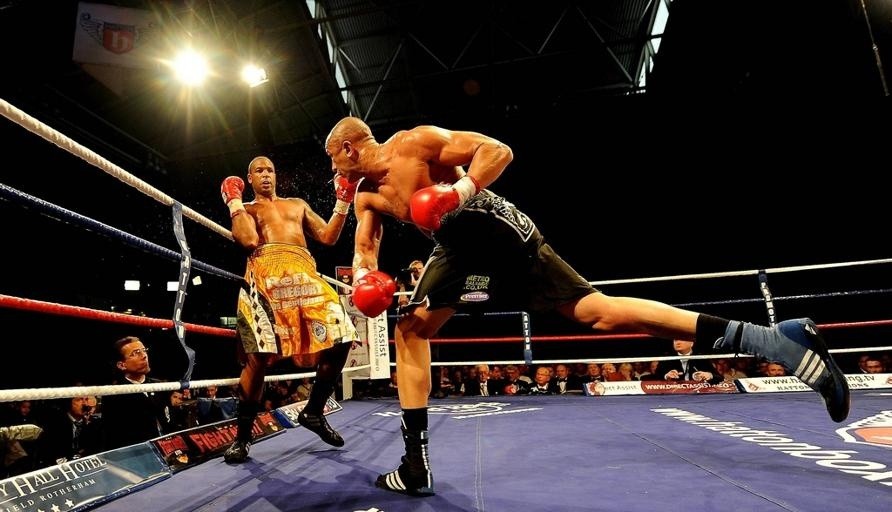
[408,175,483,233]
[349,265,399,318]
[220,174,246,217]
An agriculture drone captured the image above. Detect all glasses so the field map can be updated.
[128,347,151,357]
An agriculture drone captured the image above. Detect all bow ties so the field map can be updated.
[536,386,548,392]
[558,380,567,383]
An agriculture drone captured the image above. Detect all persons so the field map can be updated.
[100,334,174,451]
[395,257,449,400]
[652,338,725,385]
[219,154,365,463]
[352,351,892,394]
[323,116,850,496]
[0,377,315,479]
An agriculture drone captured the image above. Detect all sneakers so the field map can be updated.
[775,312,852,423]
[375,464,435,498]
[223,436,251,463]
[297,407,346,447]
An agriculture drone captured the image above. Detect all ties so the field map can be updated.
[71,419,83,437]
